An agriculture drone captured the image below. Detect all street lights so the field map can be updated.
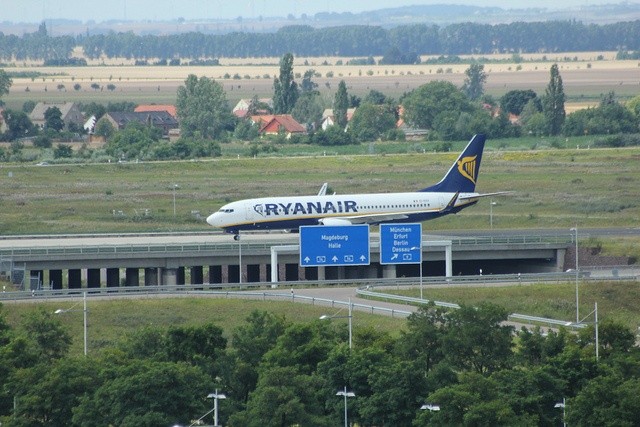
[554,396,568,426]
[317,297,354,352]
[566,301,599,361]
[489,198,499,229]
[421,403,439,414]
[53,291,87,354]
[205,387,226,426]
[335,385,355,427]
[568,223,581,323]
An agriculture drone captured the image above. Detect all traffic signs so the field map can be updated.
[379,223,421,264]
[298,223,371,268]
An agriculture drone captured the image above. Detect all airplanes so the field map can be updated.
[206,132,517,241]
